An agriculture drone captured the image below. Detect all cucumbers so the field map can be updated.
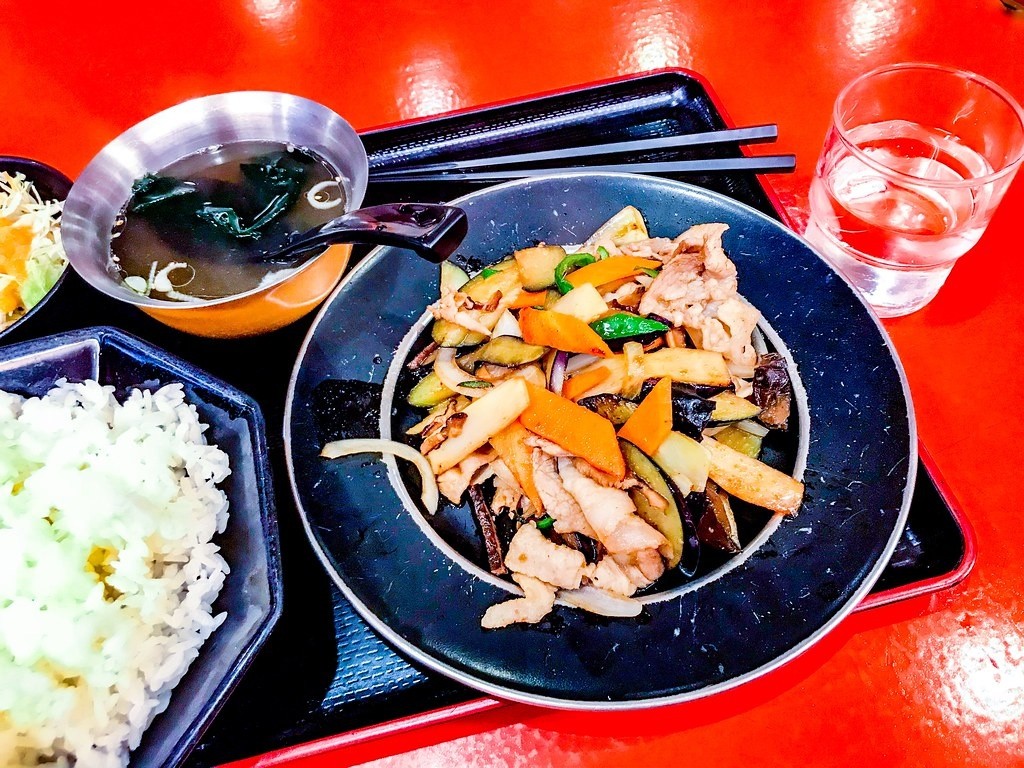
[589,307,675,341]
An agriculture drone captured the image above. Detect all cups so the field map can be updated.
[804,61,1023,318]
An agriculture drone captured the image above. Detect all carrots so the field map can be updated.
[563,252,663,291]
[489,421,544,515]
[616,378,675,458]
[510,289,616,359]
[521,377,625,475]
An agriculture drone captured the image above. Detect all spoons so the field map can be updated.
[147,175,469,266]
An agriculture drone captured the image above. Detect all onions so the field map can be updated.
[549,349,600,395]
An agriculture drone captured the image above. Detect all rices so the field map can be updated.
[0,373,236,768]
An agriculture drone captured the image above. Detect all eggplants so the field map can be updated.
[575,352,791,443]
[402,240,572,435]
[617,437,743,576]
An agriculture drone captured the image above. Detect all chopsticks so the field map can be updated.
[366,124,797,181]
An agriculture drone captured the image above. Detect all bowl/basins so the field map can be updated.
[0,156,103,349]
[60,91,370,341]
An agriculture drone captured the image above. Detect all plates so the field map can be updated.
[283,166,919,710]
[0,322,283,766]
